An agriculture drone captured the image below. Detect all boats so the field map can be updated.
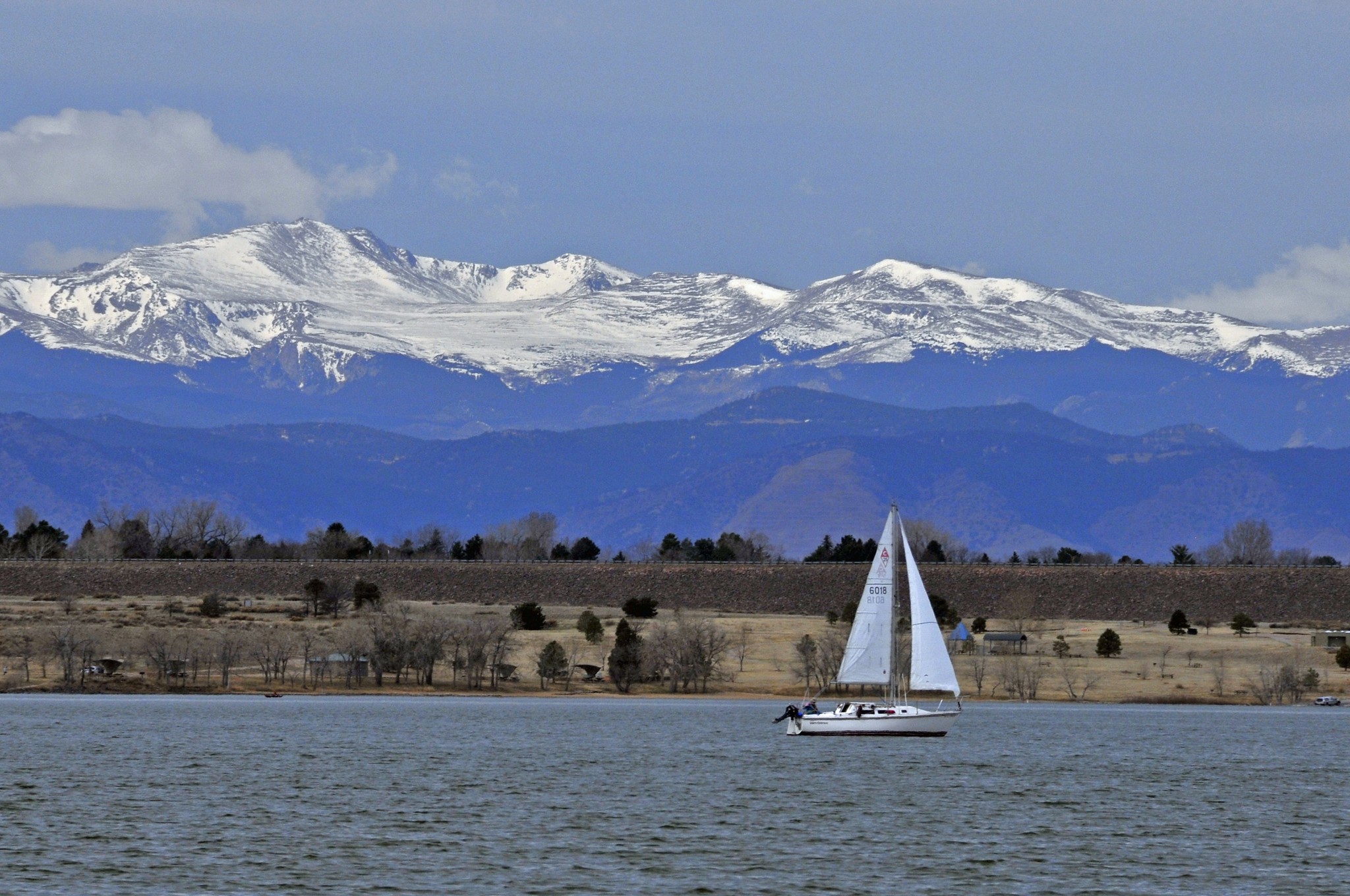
[265,694,284,698]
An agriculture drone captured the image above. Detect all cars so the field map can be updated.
[80,665,104,675]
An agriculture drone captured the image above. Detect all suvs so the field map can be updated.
[1314,696,1341,706]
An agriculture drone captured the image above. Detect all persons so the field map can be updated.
[803,698,819,715]
[3,666,7,674]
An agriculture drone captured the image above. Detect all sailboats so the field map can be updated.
[771,496,972,737]
